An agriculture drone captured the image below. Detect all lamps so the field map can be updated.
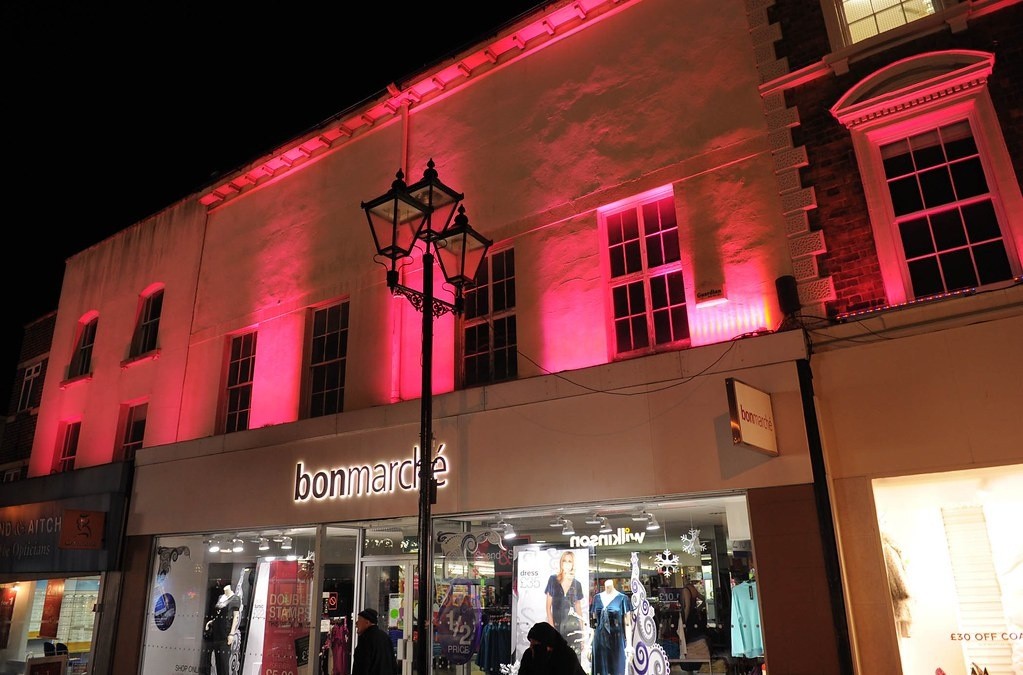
[490,512,516,540]
[232,535,245,553]
[206,537,231,553]
[250,536,270,550]
[550,514,575,536]
[631,508,660,531]
[273,535,293,549]
[585,512,613,533]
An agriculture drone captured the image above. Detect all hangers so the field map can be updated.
[746,568,756,583]
[488,615,507,624]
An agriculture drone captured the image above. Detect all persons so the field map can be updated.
[685,583,704,640]
[518,622,586,675]
[545,551,584,664]
[591,580,632,675]
[352,608,401,675]
[206,585,241,675]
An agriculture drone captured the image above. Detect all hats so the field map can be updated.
[358,608,379,625]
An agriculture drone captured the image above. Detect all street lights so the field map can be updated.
[359,155,495,674]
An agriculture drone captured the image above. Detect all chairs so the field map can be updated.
[56,643,81,672]
[43,642,55,657]
